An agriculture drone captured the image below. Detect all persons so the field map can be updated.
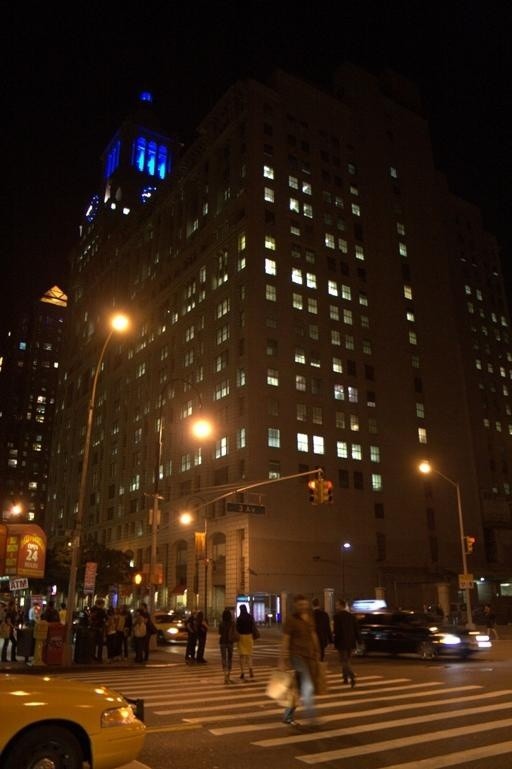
[333,599,361,688]
[196,612,207,663]
[218,609,237,685]
[185,615,197,663]
[311,598,333,661]
[1,592,157,665]
[436,603,444,617]
[280,594,321,729]
[236,605,256,679]
[483,605,499,640]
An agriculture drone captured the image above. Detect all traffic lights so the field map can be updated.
[322,481,334,505]
[308,481,321,506]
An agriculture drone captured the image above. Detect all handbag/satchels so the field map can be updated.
[231,631,239,642]
[252,624,260,640]
[311,660,327,693]
[265,669,300,708]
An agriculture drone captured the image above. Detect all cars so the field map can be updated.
[148,611,189,645]
[350,611,491,660]
[0,672,147,768]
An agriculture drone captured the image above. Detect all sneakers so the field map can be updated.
[344,677,357,689]
[240,668,253,679]
[282,718,303,730]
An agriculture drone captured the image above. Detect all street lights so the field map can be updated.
[147,380,219,621]
[56,311,130,669]
[178,495,209,626]
[417,459,477,628]
[135,574,142,610]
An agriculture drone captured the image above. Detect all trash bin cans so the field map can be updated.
[74,628,97,665]
